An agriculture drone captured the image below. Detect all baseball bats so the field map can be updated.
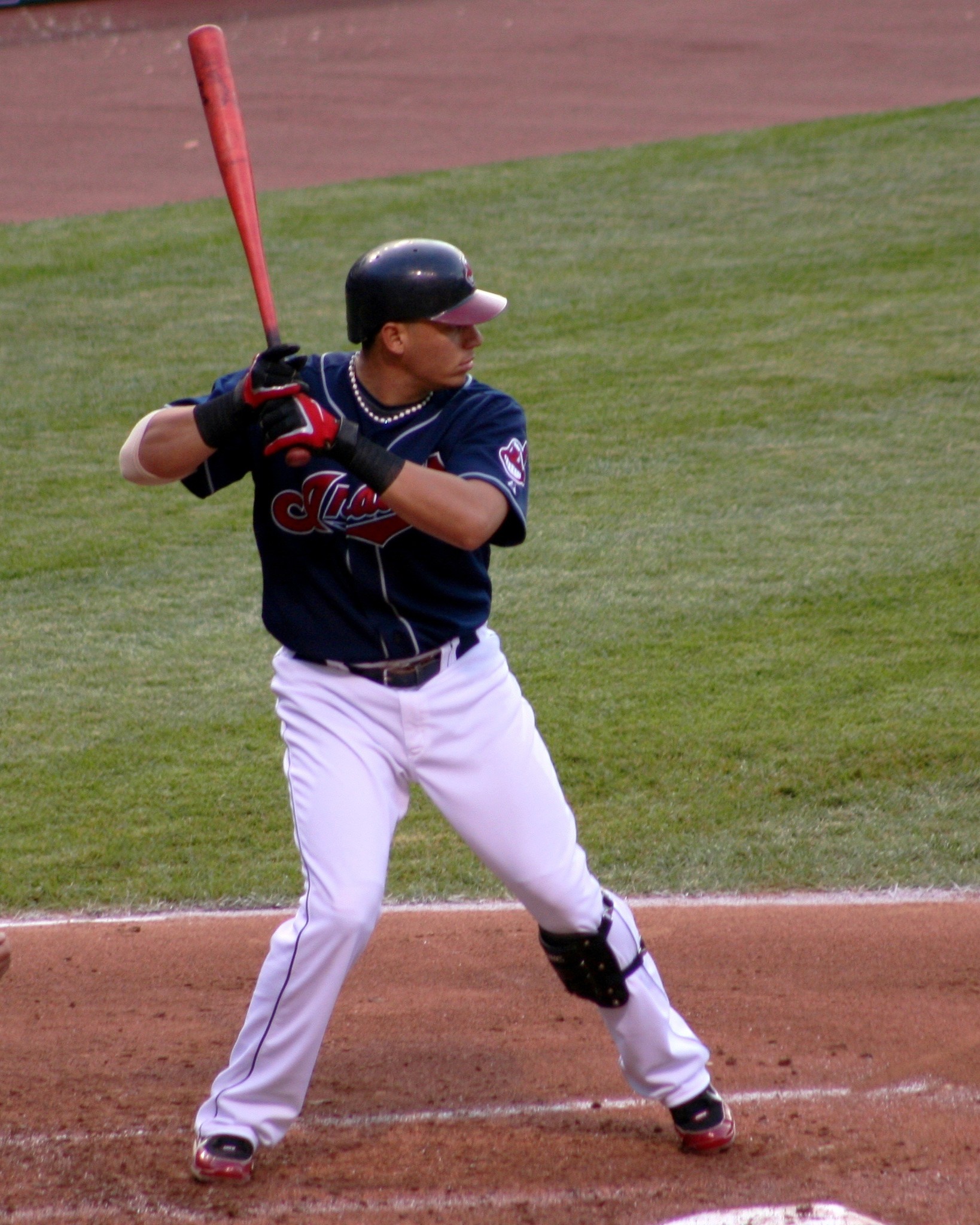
[184,22,311,465]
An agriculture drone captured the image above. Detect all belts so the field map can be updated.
[293,629,481,687]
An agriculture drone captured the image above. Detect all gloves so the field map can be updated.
[262,392,361,468]
[193,343,309,449]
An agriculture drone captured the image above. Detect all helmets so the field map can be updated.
[345,237,507,345]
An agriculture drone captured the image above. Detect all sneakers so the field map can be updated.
[191,1130,256,1185]
[669,1082,737,1155]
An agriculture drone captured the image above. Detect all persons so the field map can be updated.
[119,238,735,1188]
